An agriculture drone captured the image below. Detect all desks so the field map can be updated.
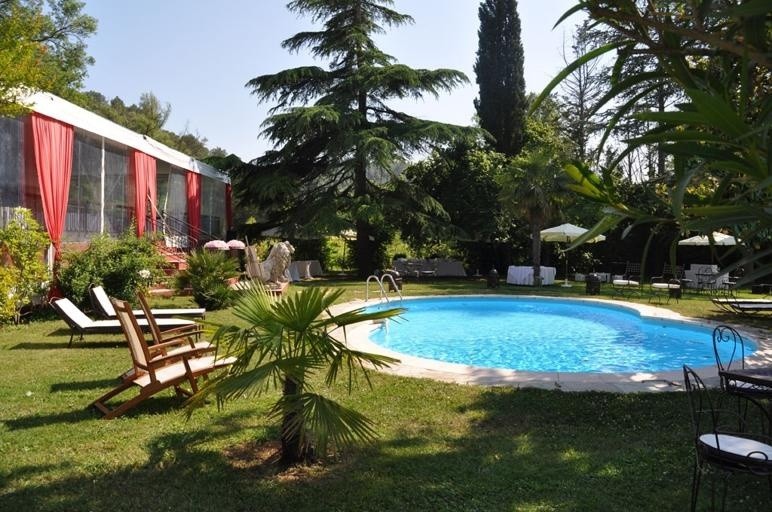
[505,265,558,286]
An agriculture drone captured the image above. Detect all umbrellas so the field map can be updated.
[527,221,606,287]
[677,227,751,266]
[312,226,375,276]
[260,218,321,261]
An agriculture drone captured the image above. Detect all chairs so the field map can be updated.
[612,266,772,512]
[49,282,242,422]
